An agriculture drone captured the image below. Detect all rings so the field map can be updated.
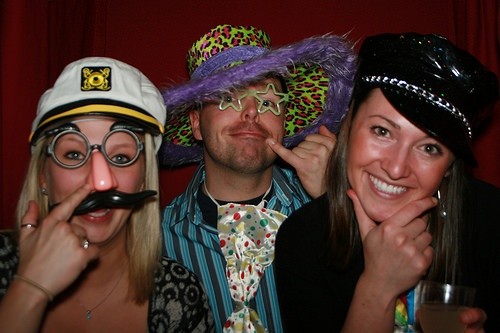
[82,239,88,250]
[21,224,37,228]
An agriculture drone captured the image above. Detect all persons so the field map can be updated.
[1,56,219,333]
[273,31,500,333]
[152,22,359,333]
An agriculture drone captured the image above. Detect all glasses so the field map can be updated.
[215,83,288,116]
[44,128,145,168]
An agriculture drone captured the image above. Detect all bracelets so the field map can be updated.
[13,273,53,300]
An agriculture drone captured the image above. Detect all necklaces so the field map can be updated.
[74,270,123,320]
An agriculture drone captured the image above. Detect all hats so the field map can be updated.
[158,25,355,164]
[353,32,500,168]
[28,56,167,150]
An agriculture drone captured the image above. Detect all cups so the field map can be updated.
[417,280,477,333]
